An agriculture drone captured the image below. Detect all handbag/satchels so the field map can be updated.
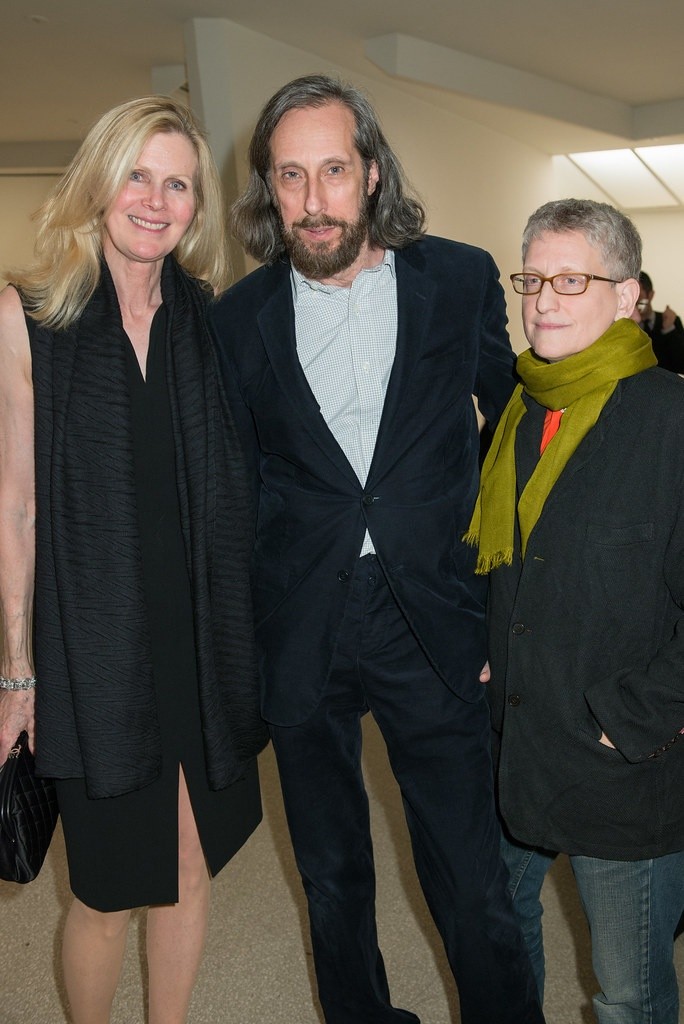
[0,730,60,884]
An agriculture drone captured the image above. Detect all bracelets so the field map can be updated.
[0,674,36,691]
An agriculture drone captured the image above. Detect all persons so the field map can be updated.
[634,270,684,375]
[0,93,266,1024]
[215,74,544,1024]
[463,199,684,1024]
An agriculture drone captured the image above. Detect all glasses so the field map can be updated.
[510,273,622,295]
